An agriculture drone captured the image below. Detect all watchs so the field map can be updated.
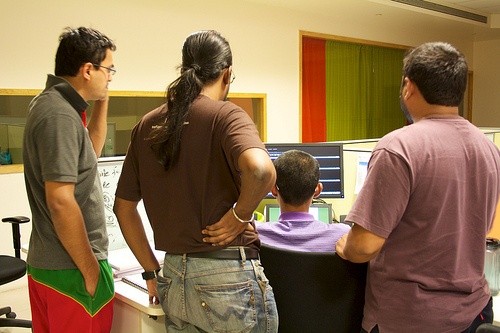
[141,268,160,280]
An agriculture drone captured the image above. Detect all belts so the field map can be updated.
[167,248,259,260]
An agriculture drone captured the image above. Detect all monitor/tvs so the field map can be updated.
[263,143,345,199]
[265,204,332,224]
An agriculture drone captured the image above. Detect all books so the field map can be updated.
[121,273,147,293]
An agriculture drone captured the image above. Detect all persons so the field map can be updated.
[22,27,116,333]
[255,149,351,255]
[113,30,279,332]
[336,41,499,332]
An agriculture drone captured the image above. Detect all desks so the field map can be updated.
[108,246,166,333]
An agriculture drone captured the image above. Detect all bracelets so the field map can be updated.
[230,202,254,224]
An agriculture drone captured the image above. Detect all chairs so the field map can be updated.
[0,215,32,328]
[260,243,368,333]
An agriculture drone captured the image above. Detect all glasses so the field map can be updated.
[230,70,235,83]
[93,64,117,75]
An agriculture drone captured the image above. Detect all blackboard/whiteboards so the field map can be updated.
[92,156,157,253]
